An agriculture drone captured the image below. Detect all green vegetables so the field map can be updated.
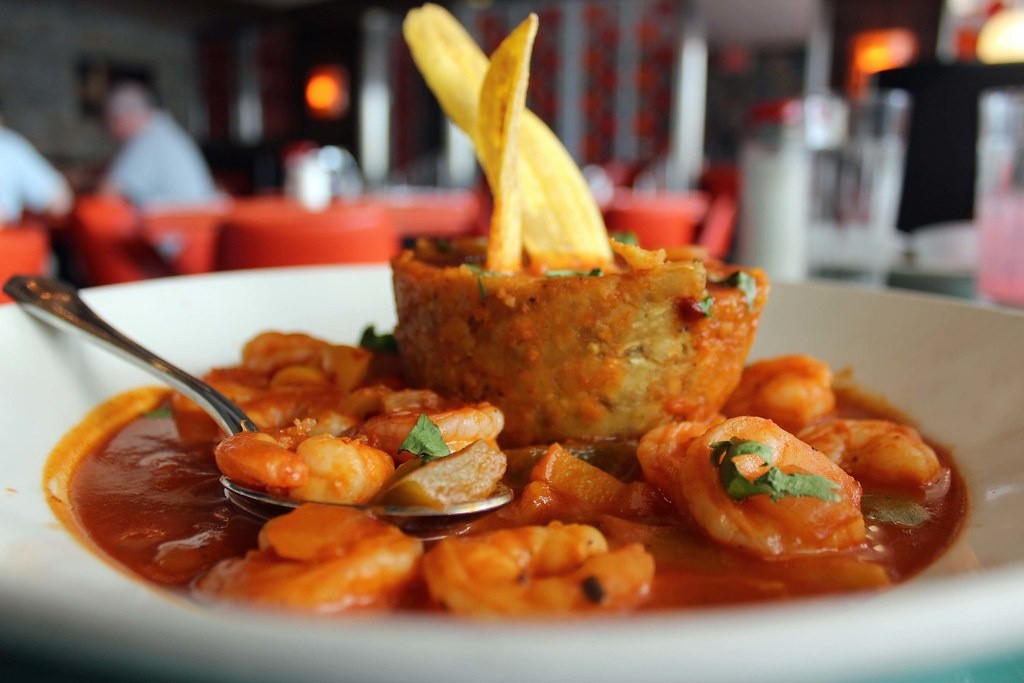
[358,239,848,503]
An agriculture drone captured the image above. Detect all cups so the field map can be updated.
[973,88,1024,306]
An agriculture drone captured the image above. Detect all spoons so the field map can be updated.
[3,275,516,534]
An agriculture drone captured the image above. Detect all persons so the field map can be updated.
[0,126,76,281]
[92,80,219,217]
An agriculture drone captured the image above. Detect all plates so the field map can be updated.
[0,263,1024,683]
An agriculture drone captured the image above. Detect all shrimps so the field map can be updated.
[170,329,943,615]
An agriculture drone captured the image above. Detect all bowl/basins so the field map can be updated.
[0,186,741,267]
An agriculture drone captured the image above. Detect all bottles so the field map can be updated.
[736,88,915,278]
